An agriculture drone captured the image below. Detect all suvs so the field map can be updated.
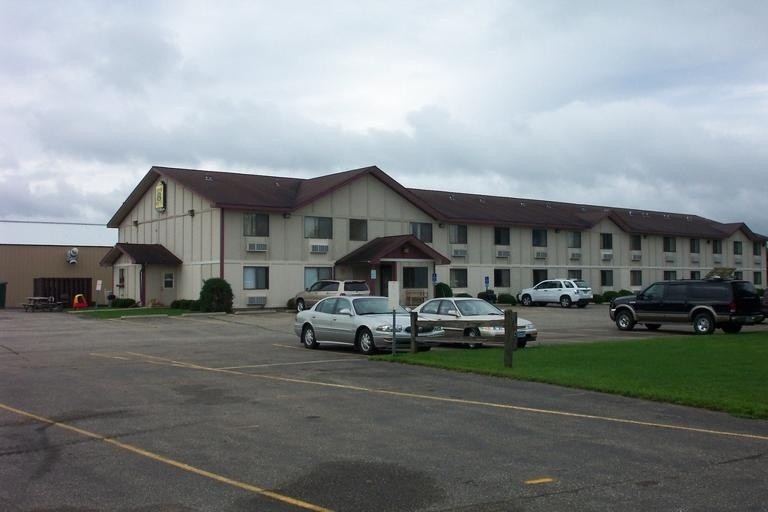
[292,280,370,312]
[519,279,593,309]
[610,280,761,336]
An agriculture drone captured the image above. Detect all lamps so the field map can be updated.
[439,222,445,228]
[132,217,138,226]
[188,209,194,216]
[283,213,291,218]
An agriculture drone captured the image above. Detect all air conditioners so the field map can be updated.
[666,256,676,261]
[753,257,762,262]
[246,242,268,252]
[690,257,700,262]
[534,250,549,258]
[451,249,468,257]
[712,257,722,262]
[247,296,267,305]
[631,254,643,261]
[496,250,511,258]
[310,244,328,252]
[734,258,743,262]
[569,252,583,260]
[600,252,614,260]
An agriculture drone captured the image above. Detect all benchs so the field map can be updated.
[22,304,35,307]
[41,302,63,305]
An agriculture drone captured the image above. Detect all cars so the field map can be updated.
[294,296,445,354]
[410,297,537,349]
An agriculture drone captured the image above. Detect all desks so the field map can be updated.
[25,296,54,312]
